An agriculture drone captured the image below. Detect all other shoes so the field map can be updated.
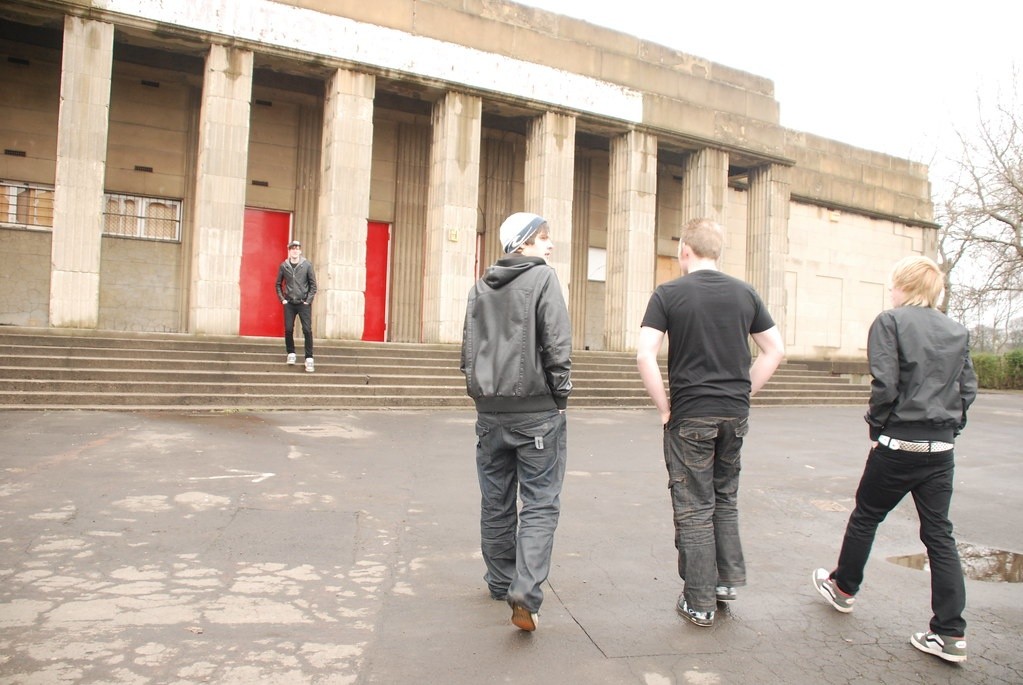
[286,352,296,364]
[509,595,539,630]
[305,357,315,371]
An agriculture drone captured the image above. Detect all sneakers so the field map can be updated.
[910,630,967,662]
[716,584,737,602]
[675,591,714,627]
[812,567,855,612]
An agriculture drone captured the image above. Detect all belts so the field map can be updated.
[878,434,955,452]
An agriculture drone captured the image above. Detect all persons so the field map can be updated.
[276,240,317,373]
[460,210,573,633]
[811,256,979,663]
[636,219,786,626]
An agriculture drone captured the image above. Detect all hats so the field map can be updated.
[288,239,302,246]
[499,210,548,254]
